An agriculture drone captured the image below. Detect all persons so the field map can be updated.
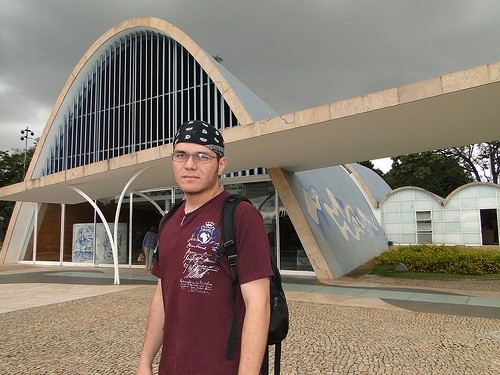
[143,223,159,272]
[136,119,274,375]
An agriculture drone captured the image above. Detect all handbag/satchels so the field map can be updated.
[137,252,144,261]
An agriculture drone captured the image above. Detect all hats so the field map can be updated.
[173,120,225,157]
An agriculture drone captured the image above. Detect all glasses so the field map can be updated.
[171,152,220,164]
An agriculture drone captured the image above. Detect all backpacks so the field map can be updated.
[158,194,289,359]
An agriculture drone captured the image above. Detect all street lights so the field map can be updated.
[20,126,34,178]
